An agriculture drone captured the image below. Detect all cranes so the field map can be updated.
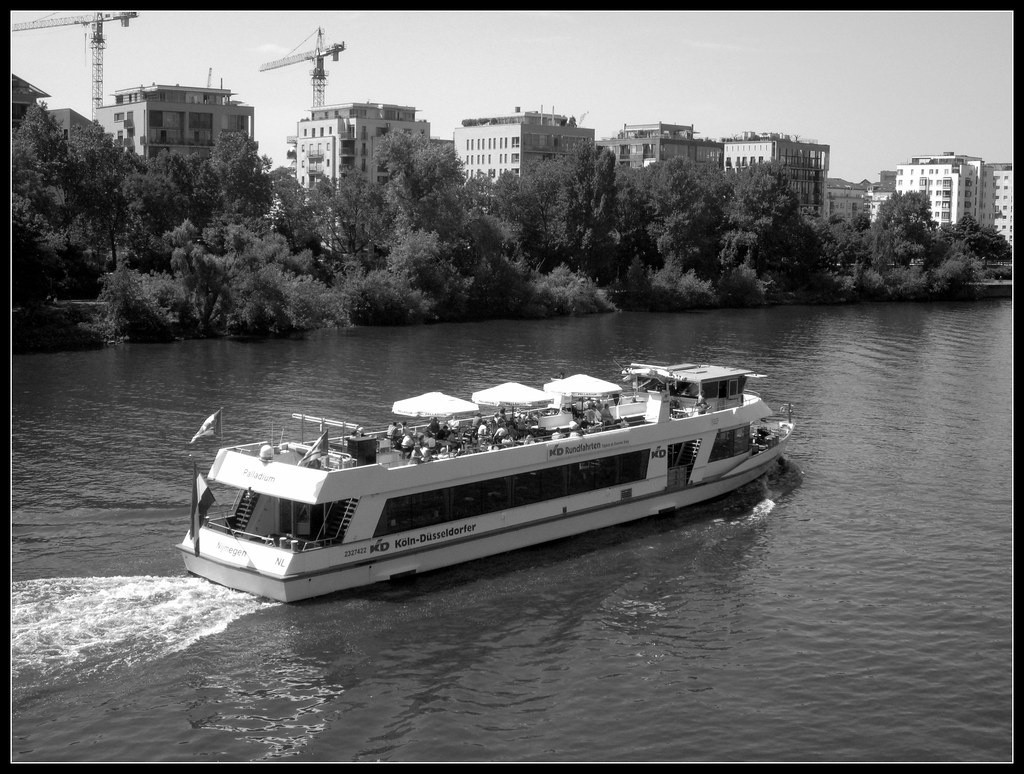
[14,11,139,121]
[258,27,346,109]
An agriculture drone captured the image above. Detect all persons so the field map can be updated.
[570,397,629,436]
[551,428,566,440]
[387,407,538,464]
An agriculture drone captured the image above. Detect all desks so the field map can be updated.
[437,440,452,447]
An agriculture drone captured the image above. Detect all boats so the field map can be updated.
[171,364,796,604]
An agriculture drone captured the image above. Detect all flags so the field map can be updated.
[297,431,329,468]
[190,410,224,442]
[188,464,216,558]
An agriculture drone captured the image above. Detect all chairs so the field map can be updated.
[385,405,603,464]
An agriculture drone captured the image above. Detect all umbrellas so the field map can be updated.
[471,381,552,419]
[543,373,623,418]
[390,391,479,435]
